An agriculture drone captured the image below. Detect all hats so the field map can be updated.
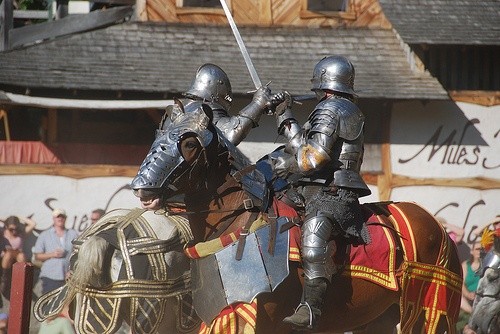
[52,208,66,217]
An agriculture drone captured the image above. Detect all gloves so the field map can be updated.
[269,91,298,135]
[237,80,272,128]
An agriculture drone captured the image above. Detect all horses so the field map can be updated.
[469,234,500,334]
[69,96,463,334]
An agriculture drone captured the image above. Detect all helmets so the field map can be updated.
[310,55,359,97]
[186,63,233,102]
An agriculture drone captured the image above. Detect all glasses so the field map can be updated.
[7,227,18,231]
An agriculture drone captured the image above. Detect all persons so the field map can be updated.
[268,55,372,330]
[0,209,500,334]
[131,63,272,209]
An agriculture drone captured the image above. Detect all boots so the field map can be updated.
[282,275,329,330]
